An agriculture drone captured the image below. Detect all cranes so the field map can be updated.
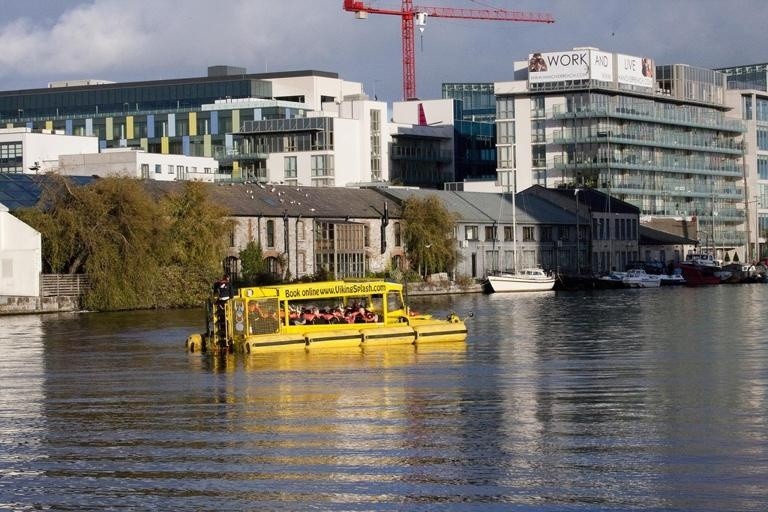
[342,1,556,101]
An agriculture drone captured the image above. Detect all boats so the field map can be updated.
[184,266,468,354]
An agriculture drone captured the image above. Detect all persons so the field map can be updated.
[212,272,234,330]
[249,298,378,326]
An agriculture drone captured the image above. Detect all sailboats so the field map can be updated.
[480,142,557,292]
[547,180,768,290]
